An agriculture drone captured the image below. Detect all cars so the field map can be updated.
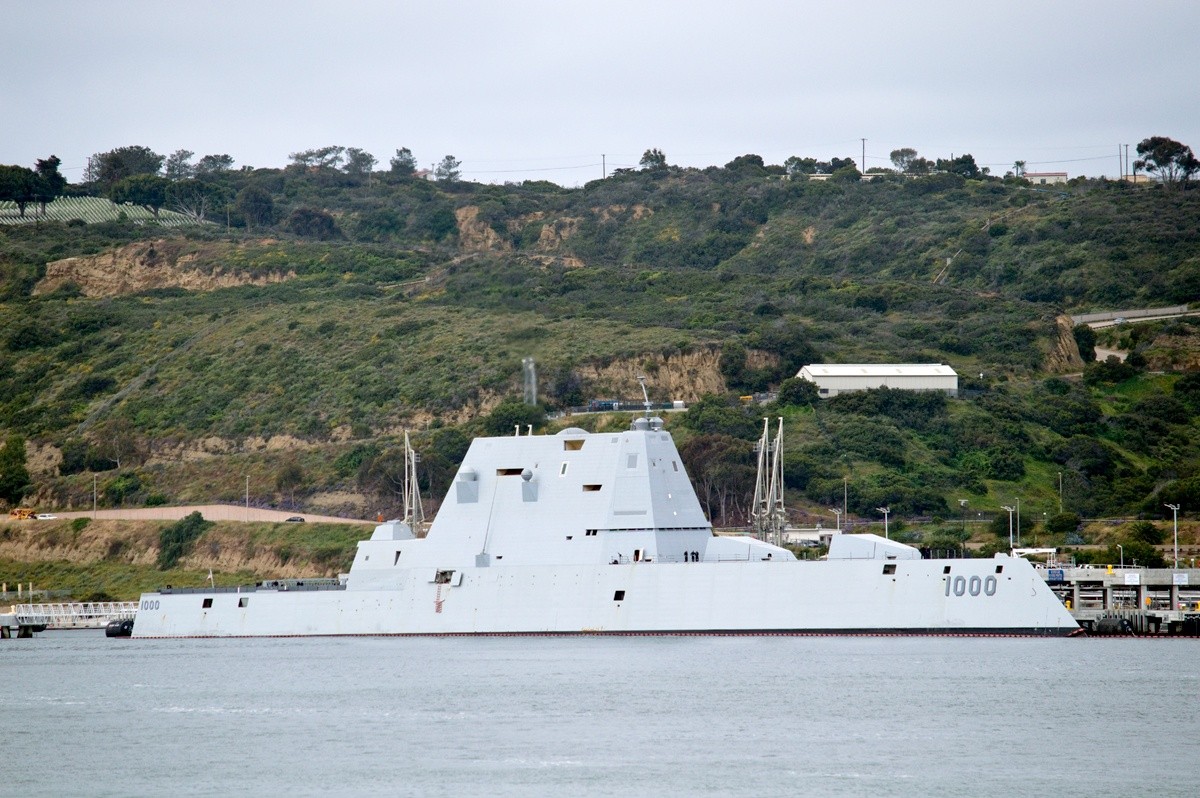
[284,516,306,522]
[37,514,58,520]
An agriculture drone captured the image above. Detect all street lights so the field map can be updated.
[1164,503,1180,568]
[246,475,251,519]
[93,473,99,521]
[1001,504,1015,550]
[828,507,842,530]
[874,505,890,538]
[1116,544,1124,568]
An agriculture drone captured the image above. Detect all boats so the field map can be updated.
[129,375,1084,638]
